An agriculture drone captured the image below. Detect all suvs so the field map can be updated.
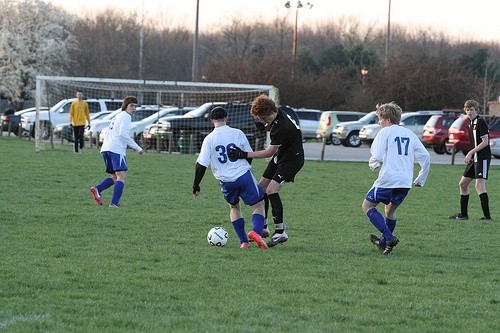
[0,96,269,153]
[420,108,467,154]
[358,110,447,148]
[330,109,380,147]
[445,113,500,161]
[287,108,324,142]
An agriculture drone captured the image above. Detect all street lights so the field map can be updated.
[284,0,314,86]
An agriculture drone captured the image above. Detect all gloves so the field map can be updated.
[228,146,248,160]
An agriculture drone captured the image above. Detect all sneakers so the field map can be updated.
[370,234,384,252]
[382,236,400,254]
[240,243,248,248]
[248,230,270,250]
[267,230,288,248]
[247,225,270,241]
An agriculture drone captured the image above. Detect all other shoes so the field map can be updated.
[90,186,103,205]
[480,217,492,221]
[109,202,120,208]
[449,214,469,220]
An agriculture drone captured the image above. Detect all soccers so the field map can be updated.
[207,227,229,246]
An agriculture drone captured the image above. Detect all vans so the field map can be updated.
[315,111,370,145]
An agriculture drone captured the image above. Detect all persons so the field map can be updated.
[192,107,270,248]
[90,96,143,208]
[69,90,91,154]
[227,94,304,248]
[361,101,430,255]
[449,100,493,220]
